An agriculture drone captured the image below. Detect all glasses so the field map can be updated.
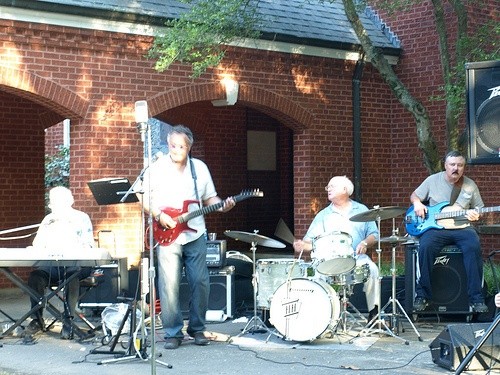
[324,185,343,191]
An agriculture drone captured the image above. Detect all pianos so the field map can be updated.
[0,245,121,349]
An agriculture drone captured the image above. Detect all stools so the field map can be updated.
[42,279,105,332]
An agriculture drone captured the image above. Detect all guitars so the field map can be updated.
[403,201,500,236]
[147,187,264,246]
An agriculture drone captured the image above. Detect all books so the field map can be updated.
[87,177,139,204]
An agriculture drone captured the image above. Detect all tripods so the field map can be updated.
[89,196,174,368]
[231,240,286,341]
[321,215,424,346]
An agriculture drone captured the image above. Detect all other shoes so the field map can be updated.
[414,298,432,310]
[164,337,180,349]
[62,325,74,339]
[474,302,488,312]
[368,319,390,329]
[194,333,208,344]
[27,319,40,334]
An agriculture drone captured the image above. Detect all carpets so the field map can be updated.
[202,316,392,350]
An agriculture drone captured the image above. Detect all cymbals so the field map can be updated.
[223,231,287,249]
[373,235,411,243]
[349,206,409,222]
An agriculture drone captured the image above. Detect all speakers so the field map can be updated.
[464,60,500,165]
[81,256,127,309]
[404,243,477,315]
[175,265,235,319]
[428,322,500,370]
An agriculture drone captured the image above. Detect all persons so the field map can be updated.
[293,175,390,330]
[136,125,236,349]
[409,151,489,312]
[27,187,97,341]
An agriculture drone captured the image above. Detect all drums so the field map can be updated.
[310,230,357,275]
[255,258,308,309]
[328,260,371,286]
[269,276,341,343]
